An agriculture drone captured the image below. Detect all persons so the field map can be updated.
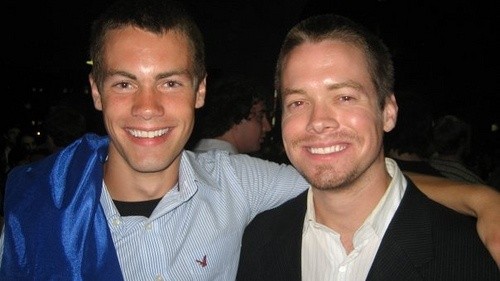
[0,9,500,281]
[235,17,500,281]
[0,76,492,188]
